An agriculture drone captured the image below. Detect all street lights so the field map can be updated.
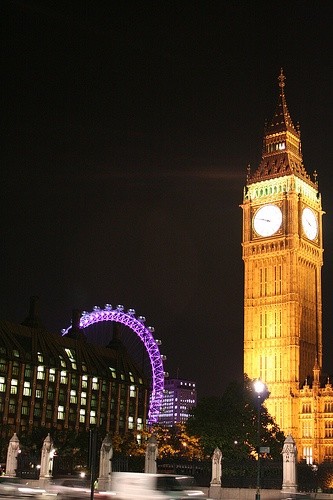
[310,464,319,492]
[254,380,265,500]
[18,449,22,478]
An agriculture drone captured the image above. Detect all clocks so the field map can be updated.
[301,207,318,241]
[250,203,283,238]
